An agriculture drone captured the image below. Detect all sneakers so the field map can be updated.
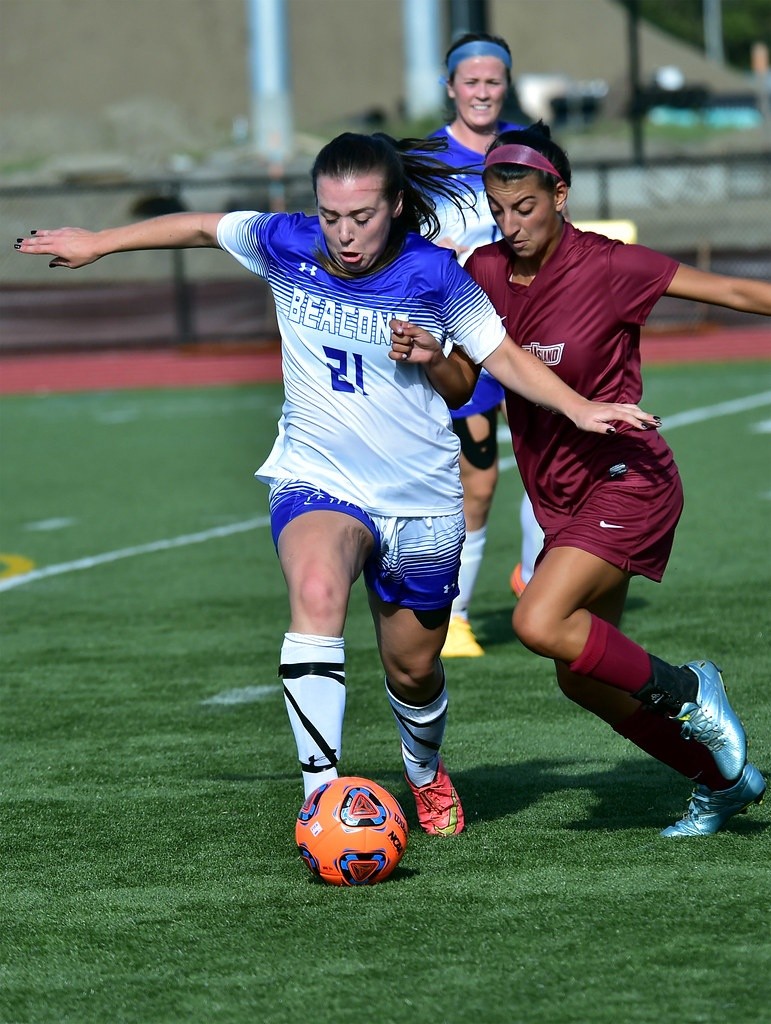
[440,613,485,659]
[510,561,528,600]
[669,658,747,781]
[405,757,465,835]
[661,764,767,837]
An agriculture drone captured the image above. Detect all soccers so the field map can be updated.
[293,777,409,886]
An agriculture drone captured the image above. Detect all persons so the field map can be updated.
[389,116,771,834]
[14,125,661,840]
[392,30,546,660]
